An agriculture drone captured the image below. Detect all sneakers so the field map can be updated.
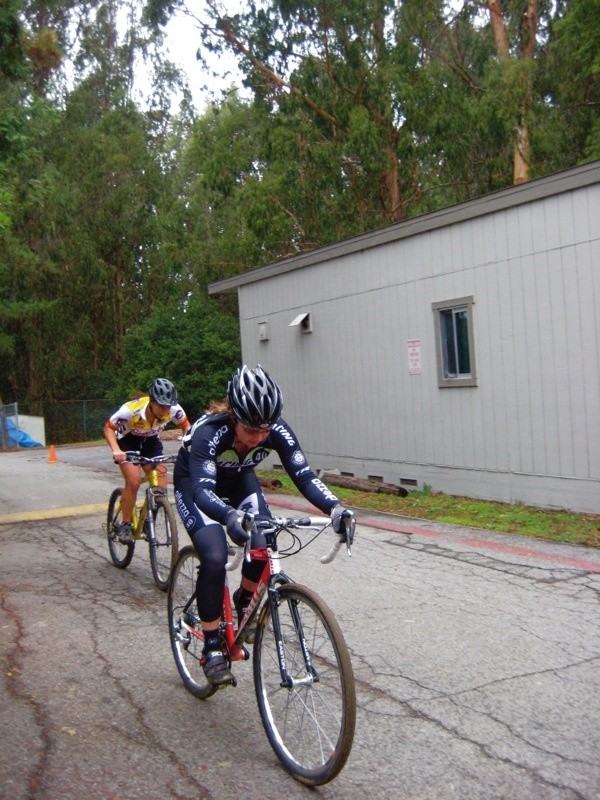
[200,648,231,684]
[120,523,134,544]
[142,520,150,536]
[233,587,260,643]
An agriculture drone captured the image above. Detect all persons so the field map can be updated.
[102,378,190,543]
[173,361,356,684]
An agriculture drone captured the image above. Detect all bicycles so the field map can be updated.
[166,509,357,790]
[107,449,180,592]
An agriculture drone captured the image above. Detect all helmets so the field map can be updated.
[148,377,178,406]
[227,364,283,430]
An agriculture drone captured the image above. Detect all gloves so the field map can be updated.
[331,504,356,546]
[222,506,258,548]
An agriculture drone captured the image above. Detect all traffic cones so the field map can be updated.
[47,444,59,464]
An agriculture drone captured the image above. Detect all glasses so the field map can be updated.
[234,416,275,433]
